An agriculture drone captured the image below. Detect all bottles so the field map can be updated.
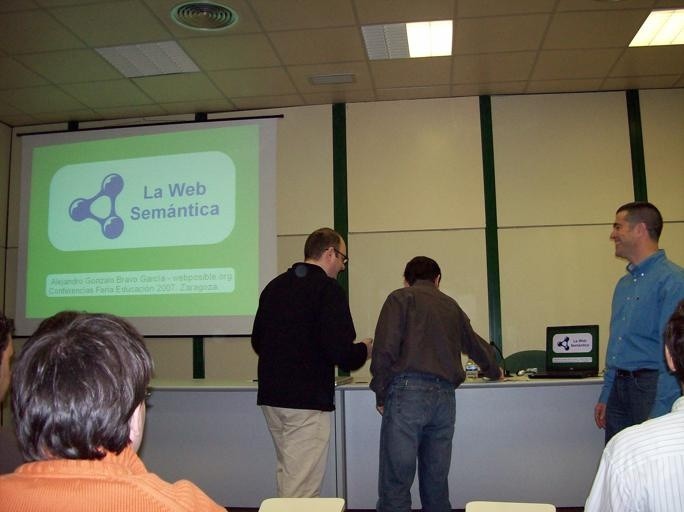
[465,355,478,378]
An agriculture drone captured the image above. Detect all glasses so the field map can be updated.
[325,249,348,263]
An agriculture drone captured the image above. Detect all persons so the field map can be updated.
[368,256,507,511]
[1,308,232,512]
[582,296,683,512]
[592,200,684,447]
[1,316,16,406]
[251,226,373,498]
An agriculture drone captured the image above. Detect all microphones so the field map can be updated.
[489,340,512,378]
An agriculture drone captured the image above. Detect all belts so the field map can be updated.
[615,369,658,377]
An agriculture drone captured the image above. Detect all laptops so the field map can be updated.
[527,324,600,379]
